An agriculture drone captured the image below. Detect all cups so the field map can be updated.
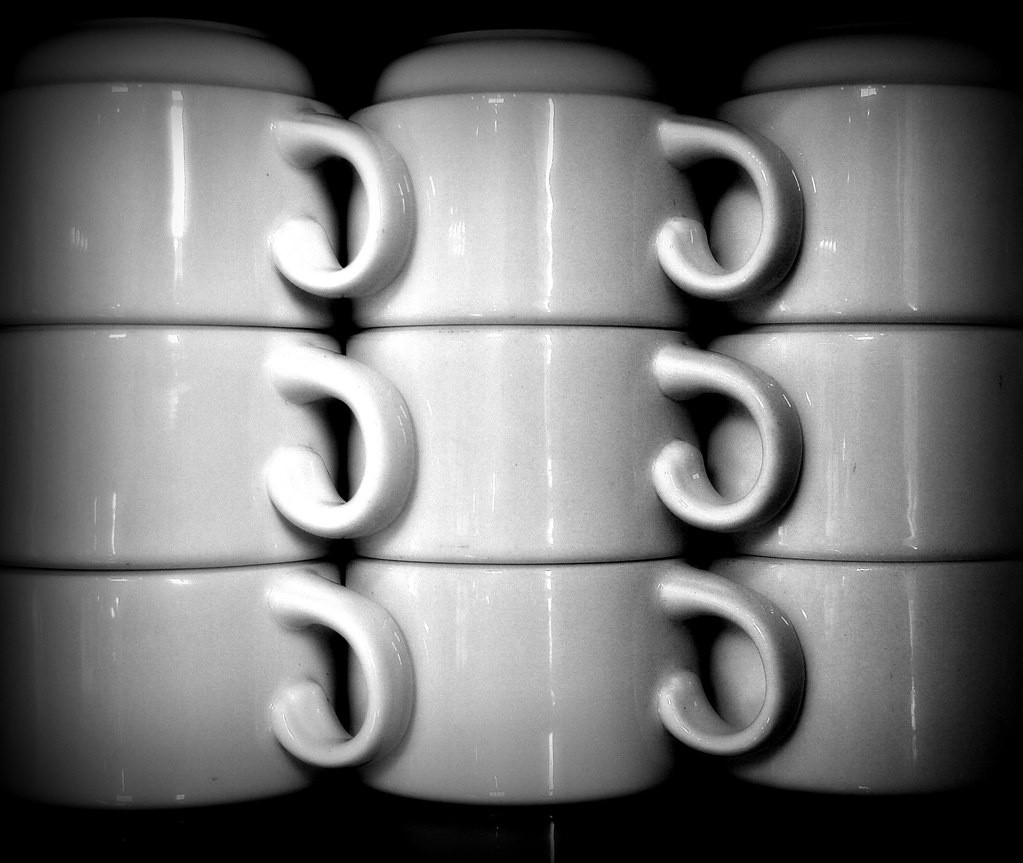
[1,30,1020,804]
